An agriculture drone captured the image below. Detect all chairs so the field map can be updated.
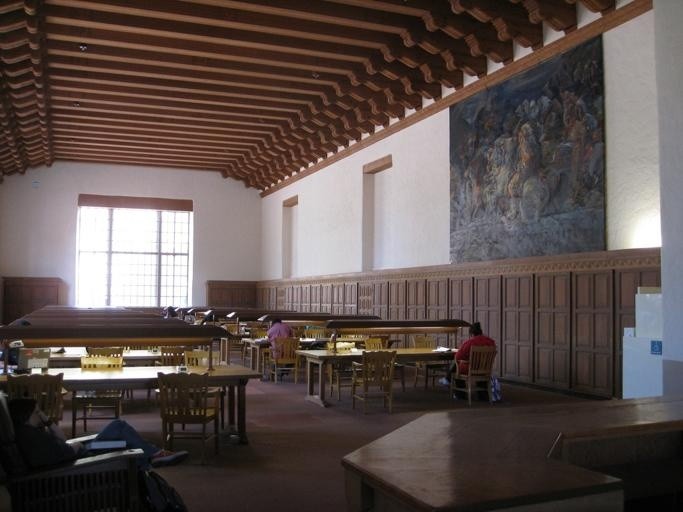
[186,350,225,428]
[87,347,124,356]
[158,373,220,457]
[226,318,497,413]
[155,347,193,366]
[70,356,124,438]
[7,374,62,423]
[0,395,146,512]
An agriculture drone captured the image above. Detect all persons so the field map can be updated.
[262,316,294,381]
[200,307,219,326]
[438,321,496,400]
[9,399,189,495]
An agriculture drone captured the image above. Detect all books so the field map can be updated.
[432,346,461,353]
[87,438,128,451]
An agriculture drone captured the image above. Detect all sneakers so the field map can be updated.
[149,448,188,465]
[438,377,449,386]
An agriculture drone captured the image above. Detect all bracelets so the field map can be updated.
[46,415,57,429]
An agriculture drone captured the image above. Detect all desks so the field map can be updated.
[48,346,185,367]
[0,366,262,444]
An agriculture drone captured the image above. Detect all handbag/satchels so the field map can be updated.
[490,373,501,401]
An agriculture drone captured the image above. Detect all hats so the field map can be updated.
[8,399,37,424]
[469,322,481,335]
